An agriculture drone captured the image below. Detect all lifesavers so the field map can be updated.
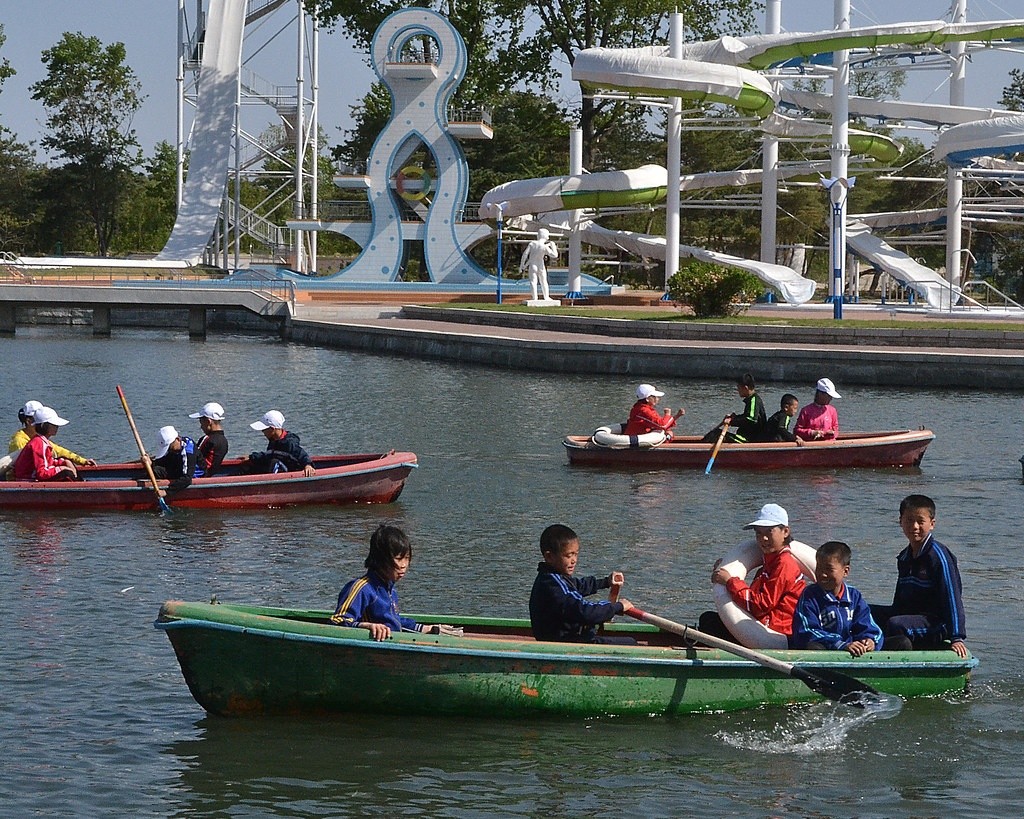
[711,537,819,650]
[594,421,666,448]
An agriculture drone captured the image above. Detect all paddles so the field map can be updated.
[115,384,175,514]
[624,608,880,710]
[705,423,729,475]
[608,573,624,603]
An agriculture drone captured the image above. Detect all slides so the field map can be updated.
[479,19,1024,309]
[7,1,251,268]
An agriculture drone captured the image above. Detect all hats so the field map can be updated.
[637,384,664,399]
[20,400,43,416]
[31,406,69,426]
[155,426,178,459]
[188,402,225,420]
[250,410,284,430]
[742,503,788,529]
[817,378,842,399]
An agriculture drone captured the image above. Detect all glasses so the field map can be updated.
[737,385,745,389]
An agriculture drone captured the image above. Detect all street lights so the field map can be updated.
[485,201,509,306]
[819,175,857,320]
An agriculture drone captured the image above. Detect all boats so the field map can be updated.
[561,420,943,482]
[152,590,982,716]
[1,449,421,507]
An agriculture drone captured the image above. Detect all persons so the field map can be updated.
[236,410,315,478]
[15,407,84,481]
[328,525,449,641]
[791,541,884,657]
[624,383,685,442]
[699,503,806,646]
[189,403,228,477]
[886,494,968,658]
[6,400,98,481]
[520,228,558,300]
[529,524,638,645]
[702,373,767,443]
[795,378,842,441]
[141,426,198,494]
[767,393,804,446]
